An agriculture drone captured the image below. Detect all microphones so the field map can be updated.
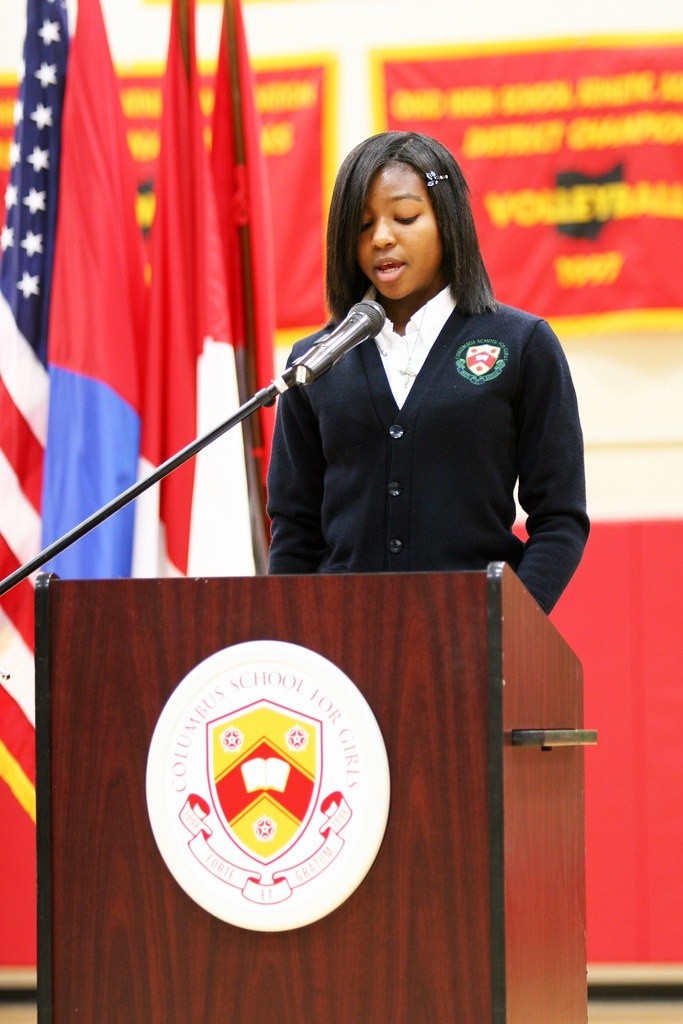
[293,303,388,386]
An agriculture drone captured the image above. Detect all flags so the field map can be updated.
[0,0,281,970]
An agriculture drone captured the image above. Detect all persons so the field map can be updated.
[265,131,590,617]
